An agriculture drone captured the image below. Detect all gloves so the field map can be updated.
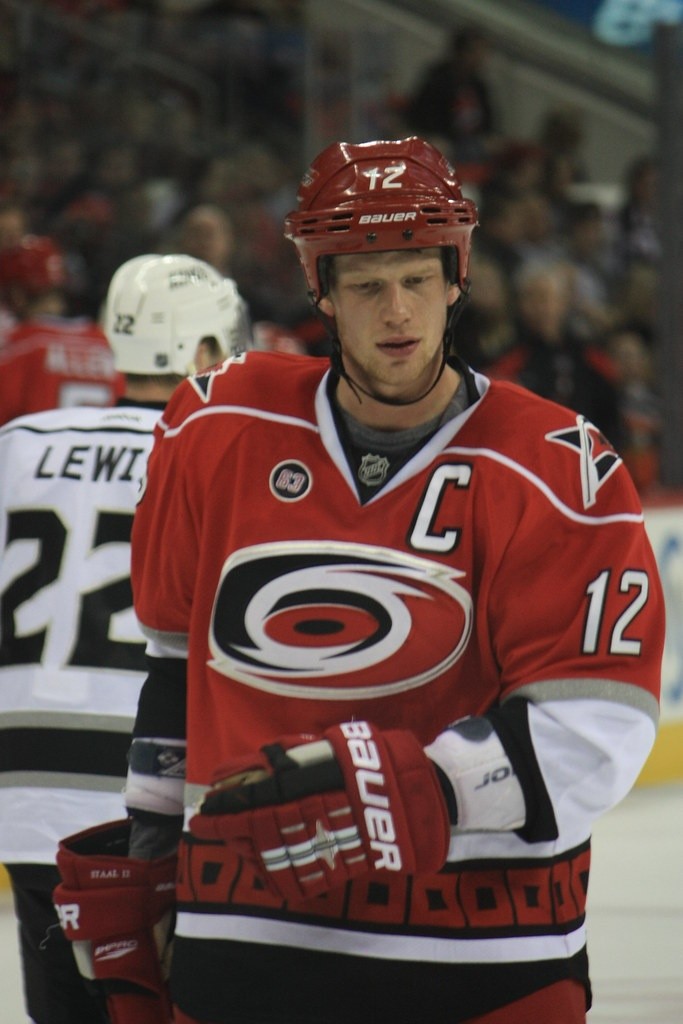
[51,816,177,1024]
[190,721,451,905]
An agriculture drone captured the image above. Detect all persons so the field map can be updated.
[0,252,245,1024]
[0,0,683,503]
[57,136,667,1024]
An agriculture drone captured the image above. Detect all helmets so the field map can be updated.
[99,252,251,379]
[283,135,479,299]
